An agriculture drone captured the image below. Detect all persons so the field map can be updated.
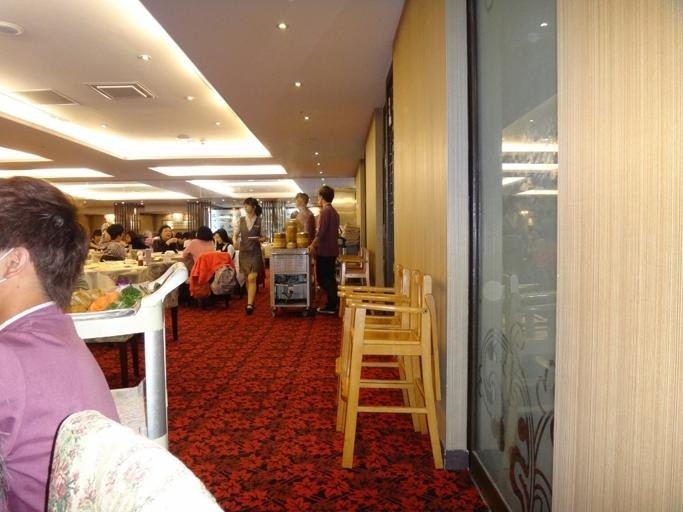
[308,186,340,315]
[1,174,121,512]
[85,224,233,303]
[293,193,315,241]
[232,198,267,315]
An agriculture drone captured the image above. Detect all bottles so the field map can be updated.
[145,249,152,266]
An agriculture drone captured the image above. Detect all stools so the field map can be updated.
[84,335,141,389]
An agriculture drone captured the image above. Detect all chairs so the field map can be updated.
[145,261,182,342]
[498,272,554,474]
[342,269,445,471]
[232,250,244,300]
[332,263,412,432]
[36,411,226,512]
[185,253,232,309]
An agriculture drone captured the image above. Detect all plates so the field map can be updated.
[248,236,259,239]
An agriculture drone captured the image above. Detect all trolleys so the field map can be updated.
[67,258,189,460]
[267,247,312,316]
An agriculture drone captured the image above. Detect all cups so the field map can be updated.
[138,256,143,265]
